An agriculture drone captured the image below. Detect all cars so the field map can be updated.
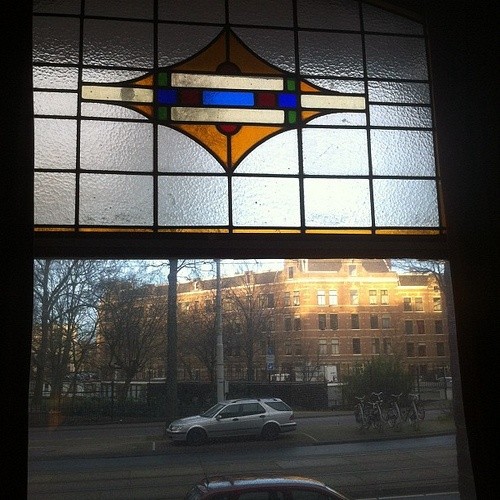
[184,472,348,500]
[166,396,298,447]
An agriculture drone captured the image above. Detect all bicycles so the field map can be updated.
[353,390,427,432]
